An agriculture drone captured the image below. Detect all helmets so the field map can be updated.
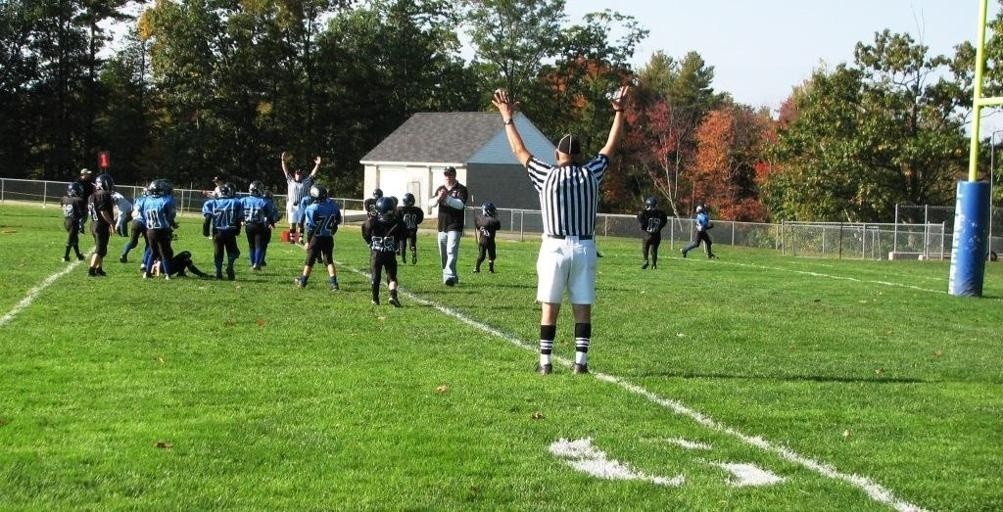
[481,201,497,217]
[212,181,237,200]
[247,179,274,201]
[65,180,84,198]
[140,176,175,198]
[308,183,329,204]
[696,204,707,214]
[94,173,115,194]
[645,196,658,211]
[403,192,415,207]
[370,188,400,216]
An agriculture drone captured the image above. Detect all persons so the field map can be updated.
[680,205,717,261]
[638,194,667,268]
[492,87,630,376]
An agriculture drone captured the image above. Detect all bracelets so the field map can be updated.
[504,118,513,125]
[614,108,625,113]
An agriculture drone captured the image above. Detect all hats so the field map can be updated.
[293,167,304,176]
[555,131,583,156]
[80,167,93,174]
[209,175,223,183]
[443,165,457,175]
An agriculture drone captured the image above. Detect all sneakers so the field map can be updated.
[680,248,688,258]
[372,297,381,307]
[571,361,591,375]
[472,267,481,274]
[64,254,71,262]
[411,253,417,265]
[295,276,307,289]
[139,263,212,281]
[226,267,235,281]
[651,262,657,270]
[298,238,305,246]
[216,272,224,280]
[287,237,296,246]
[249,261,267,271]
[401,256,407,264]
[642,263,649,269]
[533,361,553,375]
[87,266,107,277]
[444,276,459,287]
[709,253,719,259]
[76,252,86,261]
[120,253,128,264]
[489,268,496,274]
[329,283,340,292]
[388,294,402,308]
